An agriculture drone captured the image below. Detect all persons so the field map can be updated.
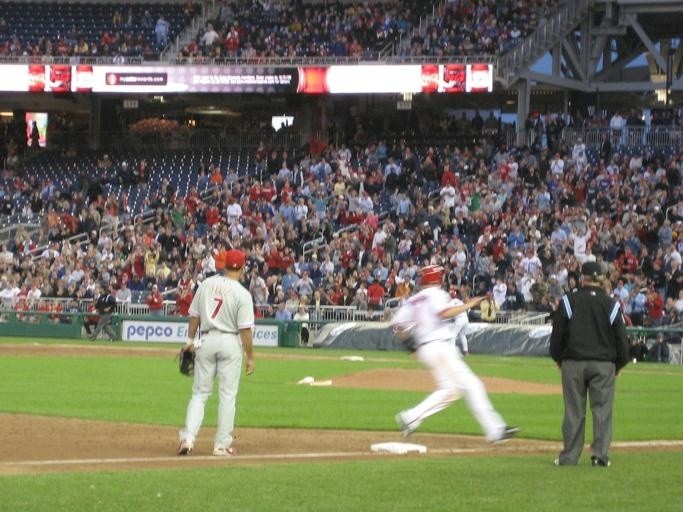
[394,265,521,445]
[1,1,682,363]
[549,261,629,466]
[175,250,256,458]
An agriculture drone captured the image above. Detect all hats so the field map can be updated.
[225,249,246,269]
[581,262,601,276]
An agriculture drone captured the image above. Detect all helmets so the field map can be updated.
[418,265,445,285]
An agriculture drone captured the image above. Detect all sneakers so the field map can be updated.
[489,426,520,445]
[212,447,238,456]
[591,455,611,466]
[176,439,194,455]
